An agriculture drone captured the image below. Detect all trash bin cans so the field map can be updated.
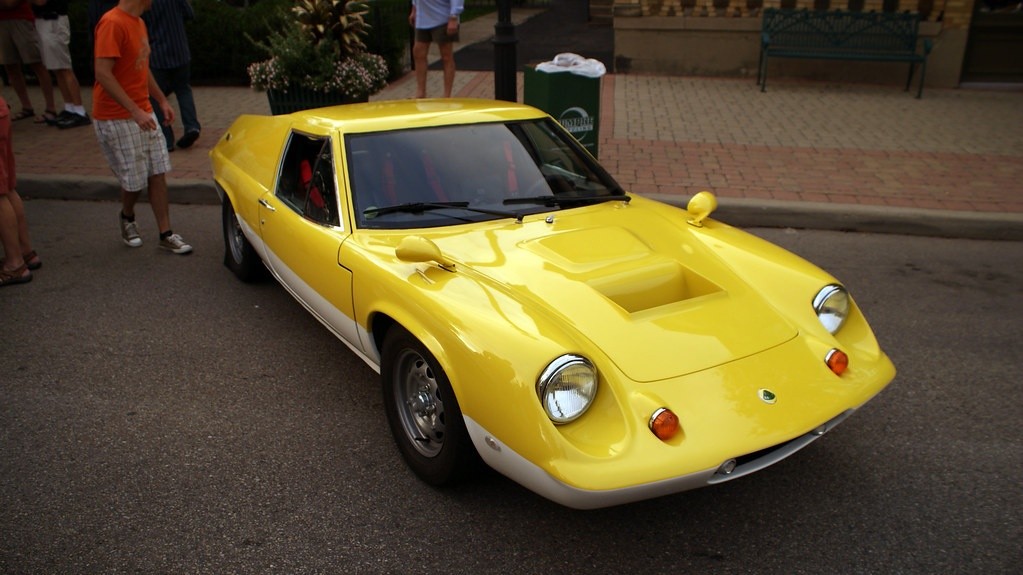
[522,65,601,164]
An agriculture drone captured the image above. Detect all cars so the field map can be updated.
[208,96,900,509]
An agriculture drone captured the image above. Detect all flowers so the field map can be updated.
[244,0,392,100]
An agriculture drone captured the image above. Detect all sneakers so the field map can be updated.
[174,129,201,149]
[157,234,191,253]
[58,112,90,128]
[119,216,143,247]
[48,108,82,125]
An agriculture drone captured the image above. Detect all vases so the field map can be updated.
[265,82,369,122]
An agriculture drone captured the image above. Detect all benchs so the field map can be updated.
[755,7,929,99]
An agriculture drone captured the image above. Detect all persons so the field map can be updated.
[408,0,465,99]
[0,0,58,123]
[30,0,93,128]
[91,0,194,254]
[140,0,201,151]
[0,95,42,286]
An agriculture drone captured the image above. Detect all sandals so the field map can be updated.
[0,265,33,285]
[32,111,56,122]
[3,250,42,270]
[11,107,34,120]
